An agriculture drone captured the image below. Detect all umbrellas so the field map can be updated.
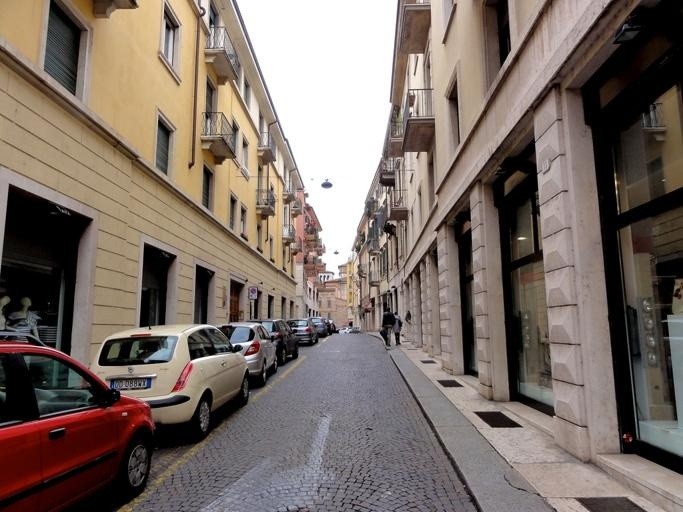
[379,326,387,345]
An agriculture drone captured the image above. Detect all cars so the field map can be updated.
[215,322,279,386]
[286,316,336,349]
[0,330,159,511]
[338,326,352,335]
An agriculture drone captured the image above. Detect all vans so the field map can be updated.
[80,316,250,442]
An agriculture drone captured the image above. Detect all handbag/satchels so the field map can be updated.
[398,320,402,327]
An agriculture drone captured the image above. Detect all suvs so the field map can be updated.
[248,319,300,365]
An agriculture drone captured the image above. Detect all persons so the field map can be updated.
[5,296,41,370]
[381,307,395,348]
[391,311,402,346]
[0,295,16,341]
